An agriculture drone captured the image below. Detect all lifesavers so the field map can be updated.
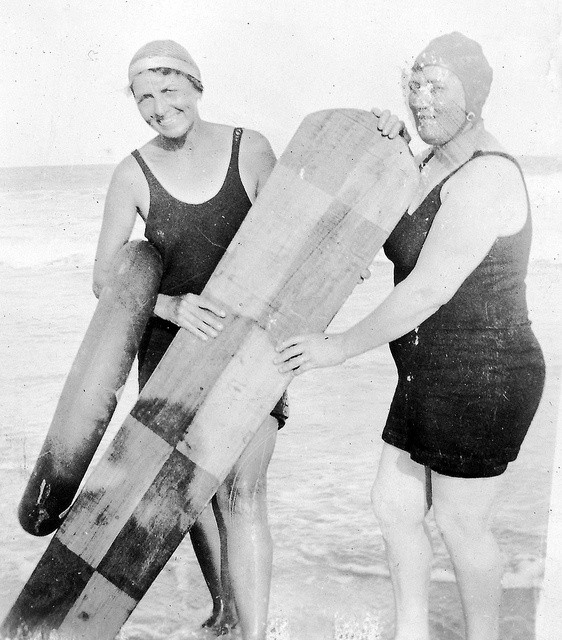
[16,239,163,537]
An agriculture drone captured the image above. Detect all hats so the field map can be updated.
[411,30,494,124]
[126,40,202,85]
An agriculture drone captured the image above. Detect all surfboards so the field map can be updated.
[0,107,421,640]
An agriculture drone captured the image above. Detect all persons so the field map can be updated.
[91,39,287,640]
[273,31,546,640]
